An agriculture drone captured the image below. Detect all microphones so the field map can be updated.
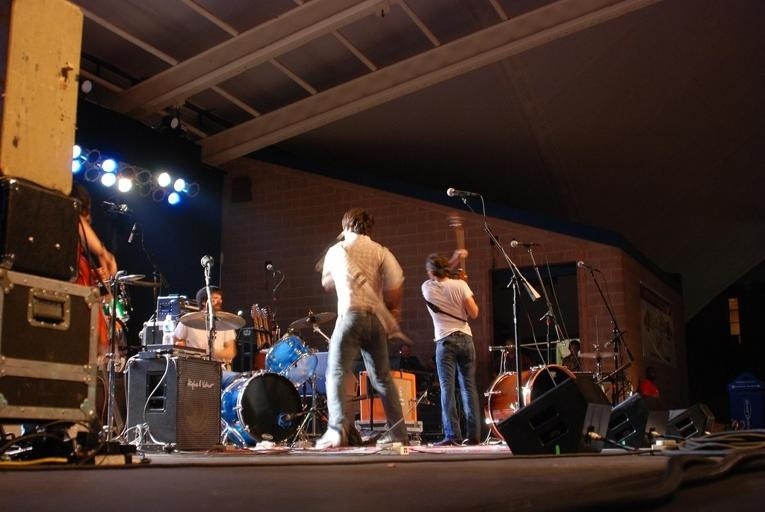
[128,222,140,245]
[202,256,212,268]
[510,239,539,248]
[447,187,477,199]
[577,260,598,272]
[237,310,244,318]
[267,264,282,277]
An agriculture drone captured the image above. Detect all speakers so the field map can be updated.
[127,358,222,451]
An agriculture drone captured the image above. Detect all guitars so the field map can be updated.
[220,371,305,449]
[248,303,277,373]
[89,253,113,304]
[264,333,319,391]
[445,209,469,284]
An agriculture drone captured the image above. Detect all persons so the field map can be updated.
[495,339,530,373]
[400,342,423,371]
[73,182,118,353]
[421,252,482,447]
[562,340,581,373]
[314,206,413,449]
[172,285,236,374]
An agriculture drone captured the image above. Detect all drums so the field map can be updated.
[482,362,578,445]
[105,313,131,375]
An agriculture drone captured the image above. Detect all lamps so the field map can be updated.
[79,79,92,94]
[162,107,178,128]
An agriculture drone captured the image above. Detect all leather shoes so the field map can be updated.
[432,437,461,447]
[315,426,345,450]
[461,437,480,446]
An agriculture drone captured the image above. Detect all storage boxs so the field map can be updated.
[0,174,82,282]
[0,0,83,194]
[0,267,101,422]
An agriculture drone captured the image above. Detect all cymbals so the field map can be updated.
[578,351,619,359]
[290,310,338,330]
[180,310,247,333]
